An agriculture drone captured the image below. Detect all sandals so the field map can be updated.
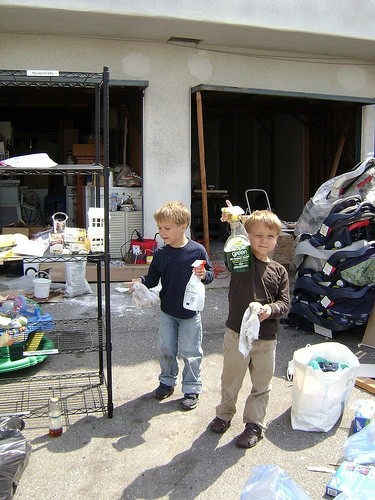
[212,417,231,433]
[237,423,262,448]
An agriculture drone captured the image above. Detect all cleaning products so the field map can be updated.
[183,260,206,311]
[220,206,253,273]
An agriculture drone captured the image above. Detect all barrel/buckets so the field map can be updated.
[33,271,51,298]
[131,239,156,264]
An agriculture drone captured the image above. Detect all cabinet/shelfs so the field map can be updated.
[0,63,114,421]
[84,184,143,231]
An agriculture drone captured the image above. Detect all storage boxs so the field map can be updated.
[64,128,79,151]
[30,225,43,234]
[2,220,31,238]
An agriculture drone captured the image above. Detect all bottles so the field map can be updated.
[48,398,62,437]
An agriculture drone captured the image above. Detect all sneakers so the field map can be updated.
[181,394,200,410]
[154,382,174,400]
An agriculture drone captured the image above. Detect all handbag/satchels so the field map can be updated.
[291,342,360,433]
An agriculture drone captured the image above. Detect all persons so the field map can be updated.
[128,200,215,411]
[209,199,291,449]
